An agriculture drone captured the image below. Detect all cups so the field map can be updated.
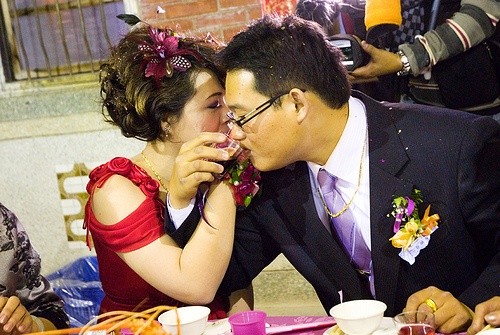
[209,132,244,181]
[228,310,267,335]
[394,311,436,335]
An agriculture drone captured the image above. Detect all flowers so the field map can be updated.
[386,187,439,251]
[137,26,190,80]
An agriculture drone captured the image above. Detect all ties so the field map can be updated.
[318,169,375,300]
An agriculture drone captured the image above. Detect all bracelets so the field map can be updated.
[30,315,45,331]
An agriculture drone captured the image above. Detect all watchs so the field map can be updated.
[396,51,410,76]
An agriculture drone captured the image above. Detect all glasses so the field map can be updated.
[227,88,306,130]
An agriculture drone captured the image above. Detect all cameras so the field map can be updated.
[327,34,365,74]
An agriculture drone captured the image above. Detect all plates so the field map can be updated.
[323,316,399,335]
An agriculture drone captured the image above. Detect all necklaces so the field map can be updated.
[140,151,168,192]
[317,140,364,218]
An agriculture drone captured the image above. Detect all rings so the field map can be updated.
[425,298,437,311]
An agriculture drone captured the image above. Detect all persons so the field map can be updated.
[84,26,254,325]
[0,203,70,335]
[163,12,500,335]
[293,0,500,124]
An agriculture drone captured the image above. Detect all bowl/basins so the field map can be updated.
[158,305,211,335]
[328,299,386,335]
